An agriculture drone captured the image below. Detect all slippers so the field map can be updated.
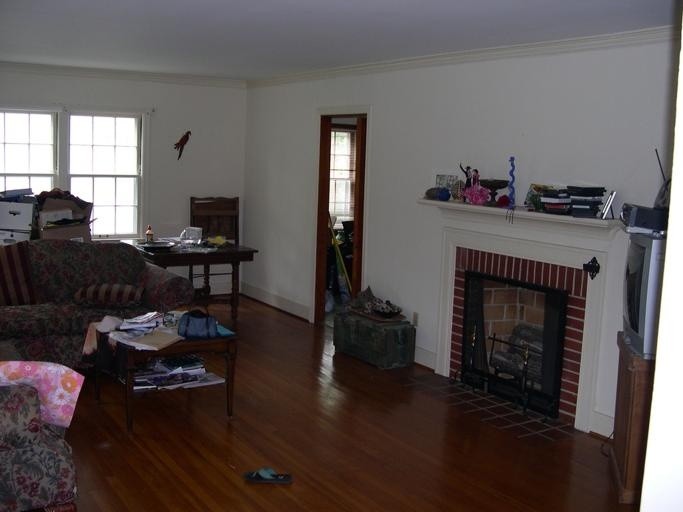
[244,467,294,484]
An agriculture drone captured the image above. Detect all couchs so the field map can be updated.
[0,241,196,393]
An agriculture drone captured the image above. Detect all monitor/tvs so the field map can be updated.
[620,231,666,361]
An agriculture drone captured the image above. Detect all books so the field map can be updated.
[529,182,608,221]
[118,311,161,332]
[130,354,226,390]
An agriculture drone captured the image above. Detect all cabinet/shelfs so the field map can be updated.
[607,331,656,510]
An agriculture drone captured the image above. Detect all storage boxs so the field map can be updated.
[0,188,93,247]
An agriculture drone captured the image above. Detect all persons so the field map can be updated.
[458,162,472,204]
[463,168,492,206]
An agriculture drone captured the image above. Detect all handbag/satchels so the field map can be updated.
[178,300,219,338]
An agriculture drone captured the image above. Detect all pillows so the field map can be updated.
[76,284,145,306]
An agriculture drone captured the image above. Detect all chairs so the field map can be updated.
[1,385,81,512]
[188,195,240,300]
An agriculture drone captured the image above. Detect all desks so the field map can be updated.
[135,243,258,322]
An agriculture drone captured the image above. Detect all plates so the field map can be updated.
[136,241,176,251]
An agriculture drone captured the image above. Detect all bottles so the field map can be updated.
[145,224,153,244]
